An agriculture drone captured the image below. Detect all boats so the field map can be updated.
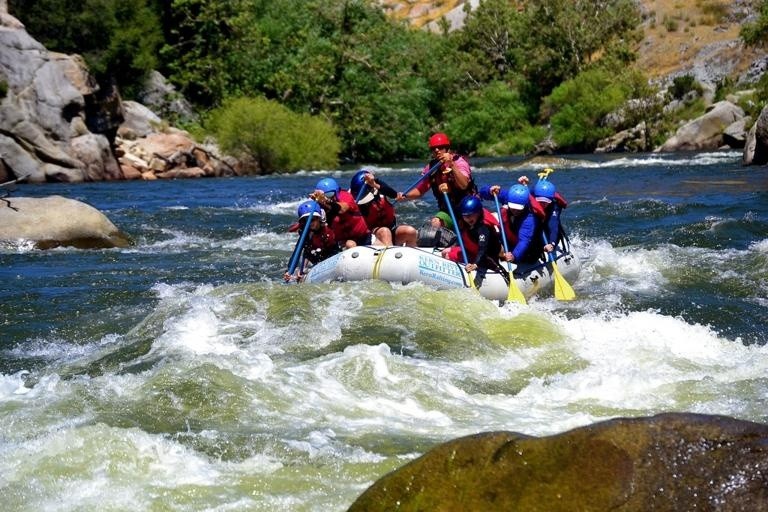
[303,244,586,307]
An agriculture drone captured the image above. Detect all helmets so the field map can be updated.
[507,184,530,211]
[297,200,326,221]
[350,170,374,196]
[461,196,483,216]
[534,180,556,204]
[430,133,450,150]
[315,177,340,197]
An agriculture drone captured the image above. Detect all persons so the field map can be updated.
[292,134,569,276]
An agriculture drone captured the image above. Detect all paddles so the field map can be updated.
[491,190,527,305]
[438,182,481,287]
[542,232,574,300]
